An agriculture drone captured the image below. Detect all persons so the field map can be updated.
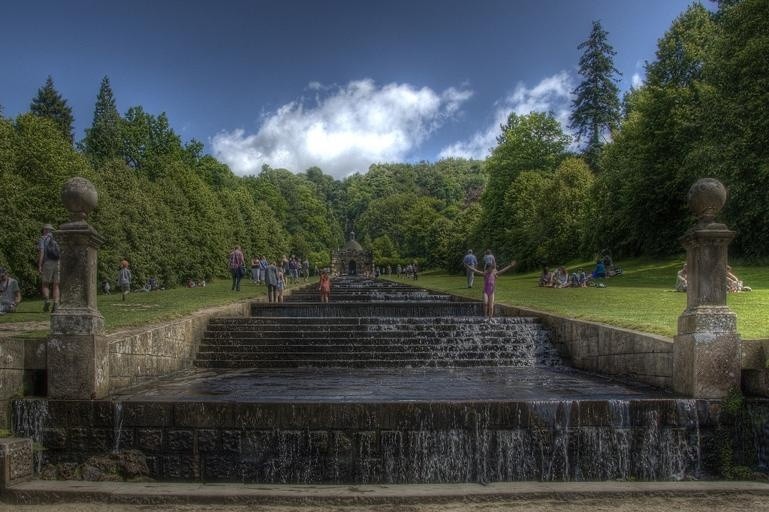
[187,279,195,288]
[537,249,615,289]
[463,260,518,321]
[38,224,61,312]
[119,260,132,301]
[463,249,478,288]
[483,248,498,272]
[229,244,417,303]
[726,264,751,292]
[675,263,688,292]
[0,267,23,317]
[104,281,110,295]
[197,277,205,287]
